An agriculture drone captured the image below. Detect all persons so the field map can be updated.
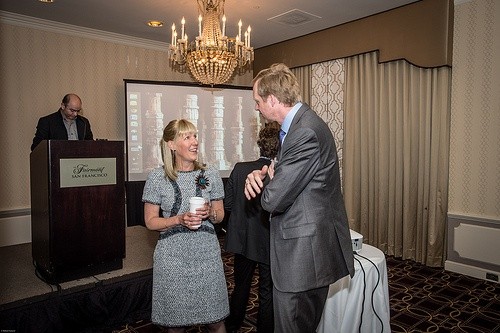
[141,120,231,333]
[222,120,281,333]
[242,64,355,333]
[31,94,96,153]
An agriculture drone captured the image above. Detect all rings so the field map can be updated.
[245,182,251,186]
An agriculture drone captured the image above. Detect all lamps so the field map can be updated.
[168,0,254,86]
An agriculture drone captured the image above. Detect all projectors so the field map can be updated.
[349,229,363,252]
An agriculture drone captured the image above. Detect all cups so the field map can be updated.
[188,197,205,228]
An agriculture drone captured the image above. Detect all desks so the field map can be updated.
[315,243,392,333]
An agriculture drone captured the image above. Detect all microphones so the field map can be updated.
[79,117,87,140]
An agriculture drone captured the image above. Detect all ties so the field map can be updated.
[276,130,286,161]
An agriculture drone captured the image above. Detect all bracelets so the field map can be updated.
[209,209,217,222]
